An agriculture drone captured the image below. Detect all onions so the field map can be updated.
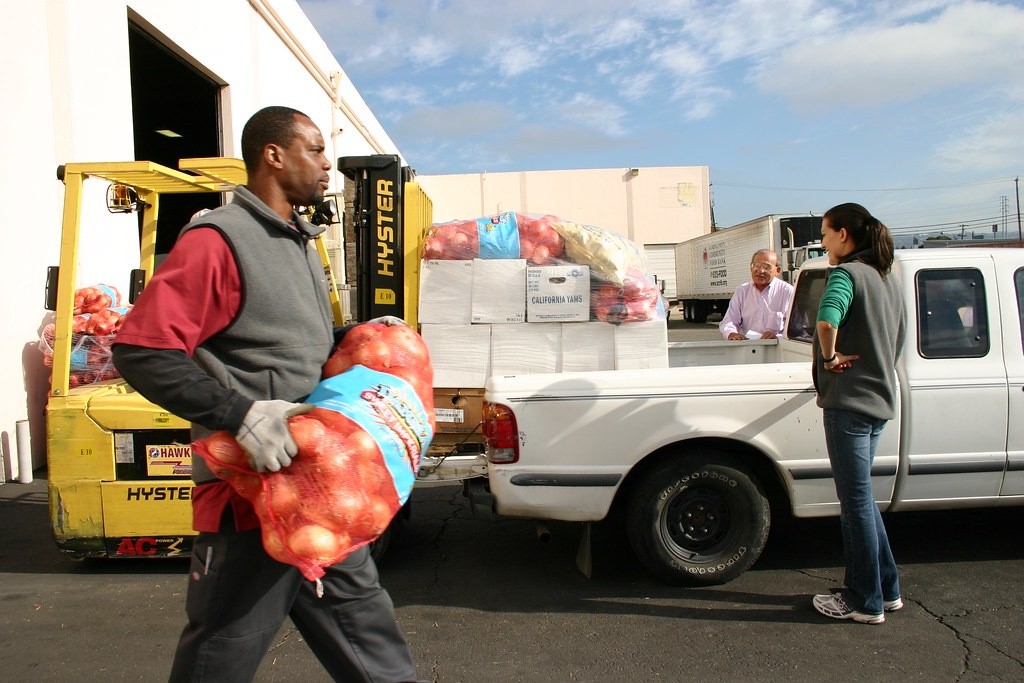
[422,213,661,324]
[36,282,138,391]
[203,319,436,567]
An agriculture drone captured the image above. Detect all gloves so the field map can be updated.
[235,398,317,474]
[369,316,408,328]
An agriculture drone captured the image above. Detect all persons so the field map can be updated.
[812,203,908,624]
[110,105,419,683]
[719,249,795,340]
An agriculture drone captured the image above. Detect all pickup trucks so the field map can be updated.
[419,245,1023,587]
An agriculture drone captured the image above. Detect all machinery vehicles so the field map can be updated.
[44,155,432,568]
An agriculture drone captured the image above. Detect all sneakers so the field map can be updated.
[883,595,904,611]
[813,592,886,624]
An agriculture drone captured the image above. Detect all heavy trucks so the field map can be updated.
[673,215,827,322]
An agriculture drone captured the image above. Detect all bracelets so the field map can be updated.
[819,354,836,362]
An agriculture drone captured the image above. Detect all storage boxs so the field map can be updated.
[418,259,669,444]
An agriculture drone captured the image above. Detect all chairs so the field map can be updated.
[928,306,973,347]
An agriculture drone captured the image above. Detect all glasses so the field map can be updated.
[752,261,779,271]
